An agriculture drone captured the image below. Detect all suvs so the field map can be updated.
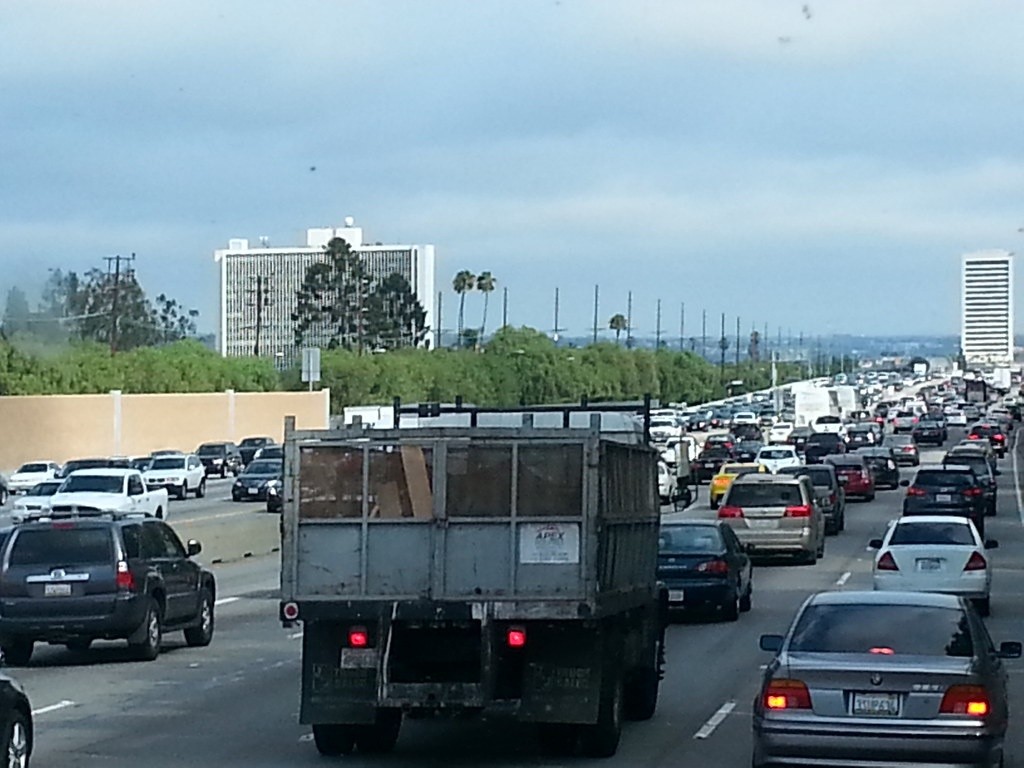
[900,464,985,541]
[194,442,243,479]
[237,437,275,467]
[0,509,216,664]
[54,457,131,481]
[717,471,826,566]
[141,452,206,501]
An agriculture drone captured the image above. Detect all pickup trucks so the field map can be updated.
[41,468,169,527]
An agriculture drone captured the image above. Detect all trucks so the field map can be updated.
[280,394,667,760]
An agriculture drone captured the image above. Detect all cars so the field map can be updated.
[0,476,8,506]
[7,461,62,495]
[11,480,68,526]
[232,458,282,502]
[778,465,849,535]
[130,456,154,471]
[657,516,756,621]
[642,361,1024,514]
[1,671,34,768]
[267,476,282,512]
[749,590,1024,768]
[869,516,999,618]
[254,445,283,461]
